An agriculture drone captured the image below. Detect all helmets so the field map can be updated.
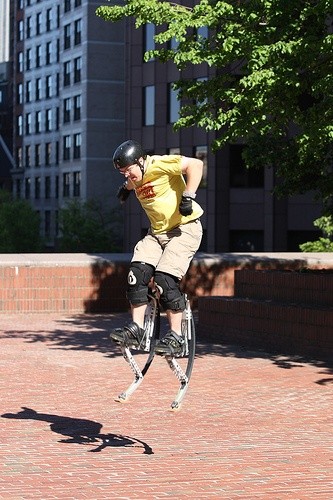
[112,139,147,170]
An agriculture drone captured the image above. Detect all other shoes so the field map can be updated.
[154,330,182,353]
[109,321,147,345]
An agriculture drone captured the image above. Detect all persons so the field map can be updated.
[109,140,204,353]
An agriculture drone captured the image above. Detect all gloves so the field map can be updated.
[116,181,133,202]
[178,191,196,217]
